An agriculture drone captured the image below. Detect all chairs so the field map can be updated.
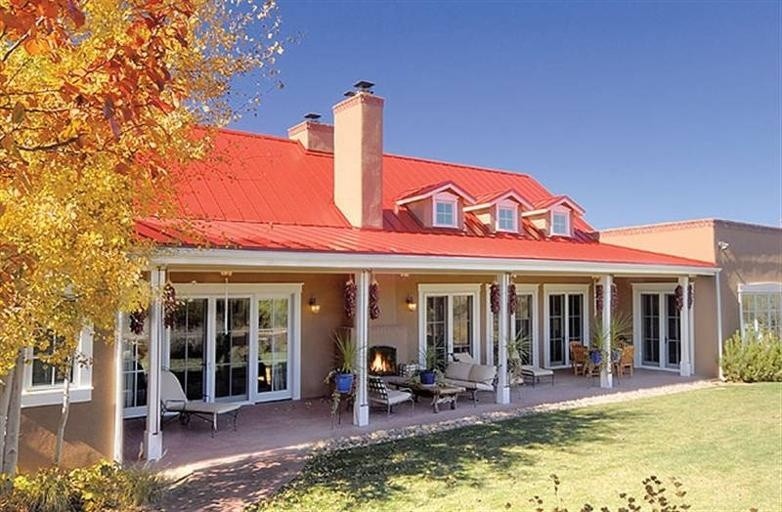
[570,340,635,378]
[505,350,555,389]
[144,368,257,439]
[452,351,497,369]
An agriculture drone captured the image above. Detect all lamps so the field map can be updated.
[309,295,321,314]
[406,296,417,312]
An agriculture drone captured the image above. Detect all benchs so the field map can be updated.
[435,361,498,408]
[347,373,415,416]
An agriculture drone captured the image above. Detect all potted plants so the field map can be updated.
[490,327,534,372]
[407,343,450,384]
[327,327,371,394]
